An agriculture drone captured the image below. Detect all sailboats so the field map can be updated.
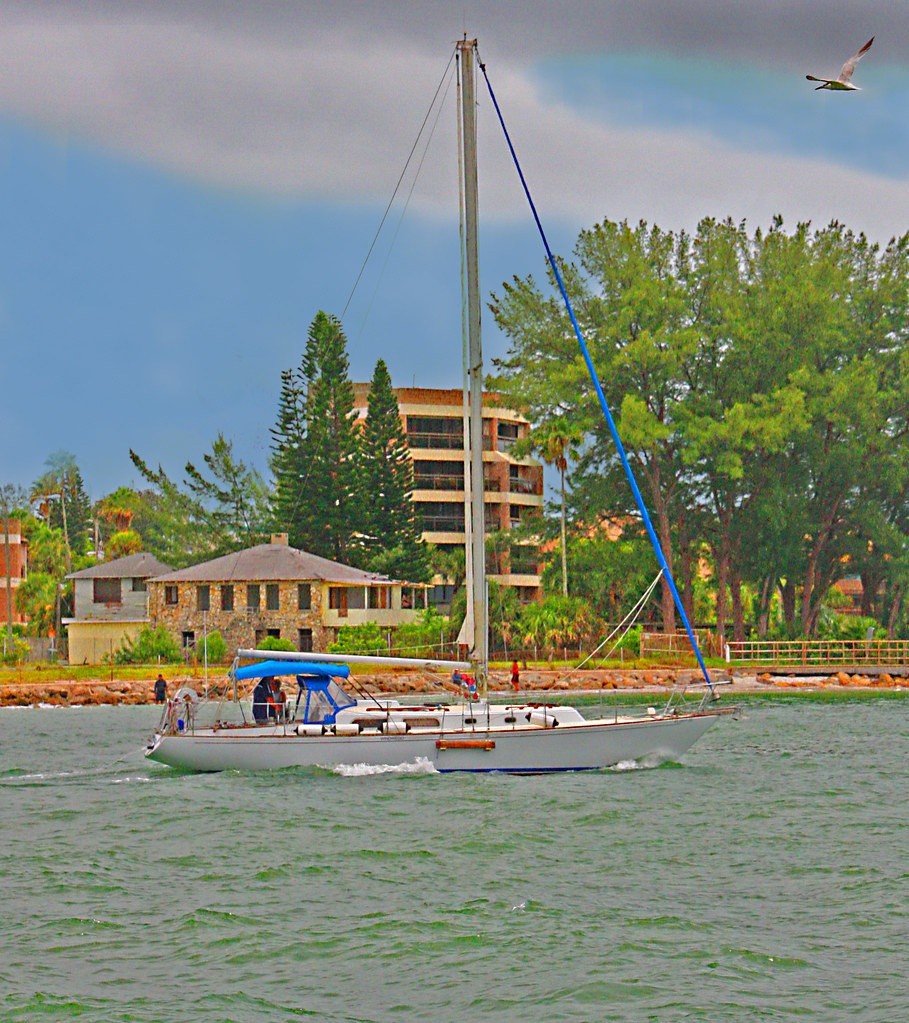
[140,28,740,774]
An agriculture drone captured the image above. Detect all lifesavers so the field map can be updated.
[174,687,199,711]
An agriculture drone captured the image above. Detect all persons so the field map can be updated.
[510,658,520,690]
[252,676,275,725]
[271,679,286,718]
[452,669,463,695]
[153,674,168,705]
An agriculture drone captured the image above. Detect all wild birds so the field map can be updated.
[805,36,875,92]
